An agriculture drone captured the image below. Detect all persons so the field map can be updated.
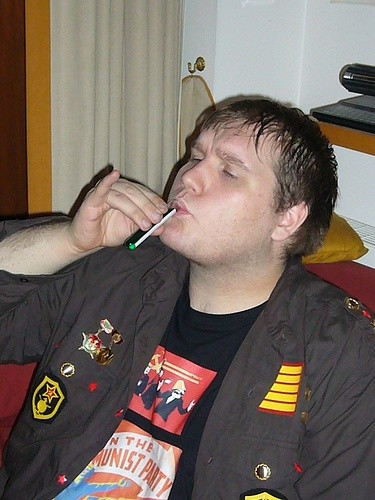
[0,98,375,500]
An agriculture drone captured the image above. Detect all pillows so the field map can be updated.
[302,212,369,264]
